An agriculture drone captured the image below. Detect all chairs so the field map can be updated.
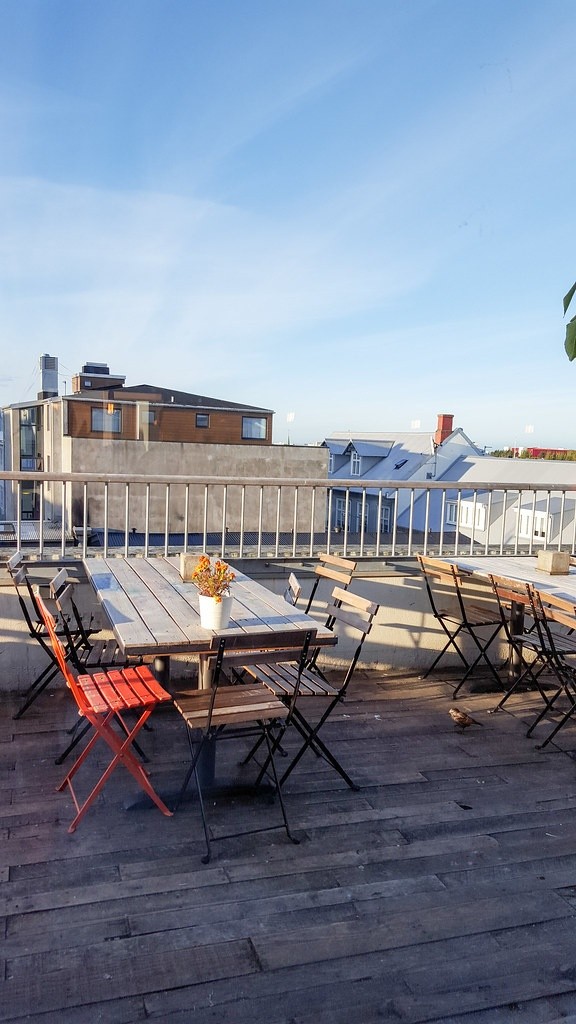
[533,588,575,750]
[172,627,320,863]
[5,549,172,833]
[485,573,576,740]
[416,554,513,699]
[211,554,380,807]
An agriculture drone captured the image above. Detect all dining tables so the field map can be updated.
[429,557,576,695]
[81,552,339,812]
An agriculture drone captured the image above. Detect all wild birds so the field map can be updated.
[447,708,484,735]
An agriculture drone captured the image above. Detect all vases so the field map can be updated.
[197,591,234,630]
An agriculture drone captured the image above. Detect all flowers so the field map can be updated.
[192,555,236,603]
